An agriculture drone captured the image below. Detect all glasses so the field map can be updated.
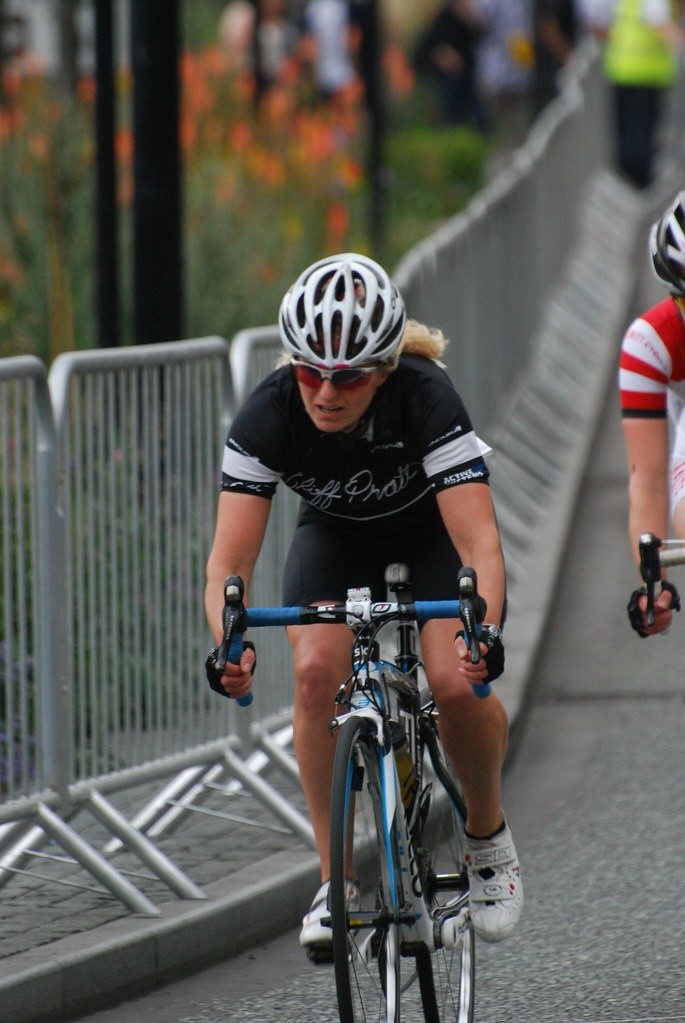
[290,355,391,391]
[671,292,685,310]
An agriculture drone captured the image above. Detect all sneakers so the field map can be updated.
[463,806,524,944]
[299,878,360,945]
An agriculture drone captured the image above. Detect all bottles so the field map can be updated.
[388,720,419,823]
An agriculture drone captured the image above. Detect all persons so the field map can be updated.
[619,188,685,639]
[221,1,684,185]
[204,252,523,967]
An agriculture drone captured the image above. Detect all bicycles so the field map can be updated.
[212,561,474,1023]
[635,533,684,627]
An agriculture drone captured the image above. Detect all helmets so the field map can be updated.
[649,191,685,293]
[278,253,407,369]
